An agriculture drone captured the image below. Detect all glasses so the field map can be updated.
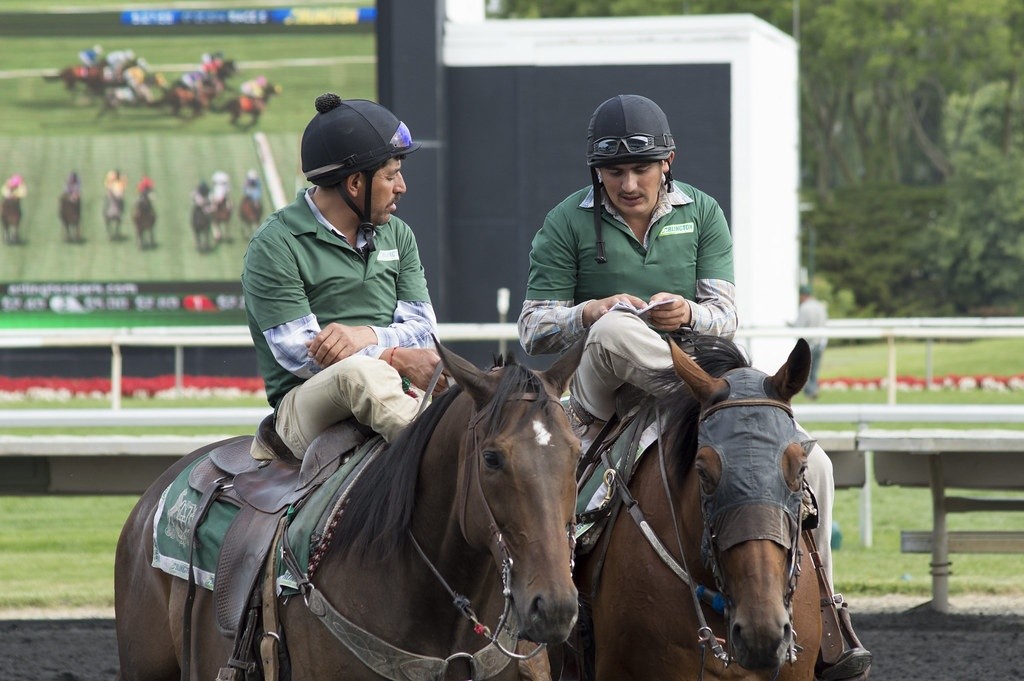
[388,120,414,152]
[591,131,657,156]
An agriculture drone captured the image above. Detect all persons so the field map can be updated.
[0,44,277,214]
[518,95,871,680]
[241,93,453,463]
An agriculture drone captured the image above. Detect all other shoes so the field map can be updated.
[814,645,872,681]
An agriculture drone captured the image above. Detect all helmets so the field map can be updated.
[300,92,423,188]
[586,94,677,167]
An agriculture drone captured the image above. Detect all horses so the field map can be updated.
[42,57,283,132]
[1,185,263,255]
[114,333,823,681]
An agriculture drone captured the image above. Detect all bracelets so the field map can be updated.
[390,346,398,366]
